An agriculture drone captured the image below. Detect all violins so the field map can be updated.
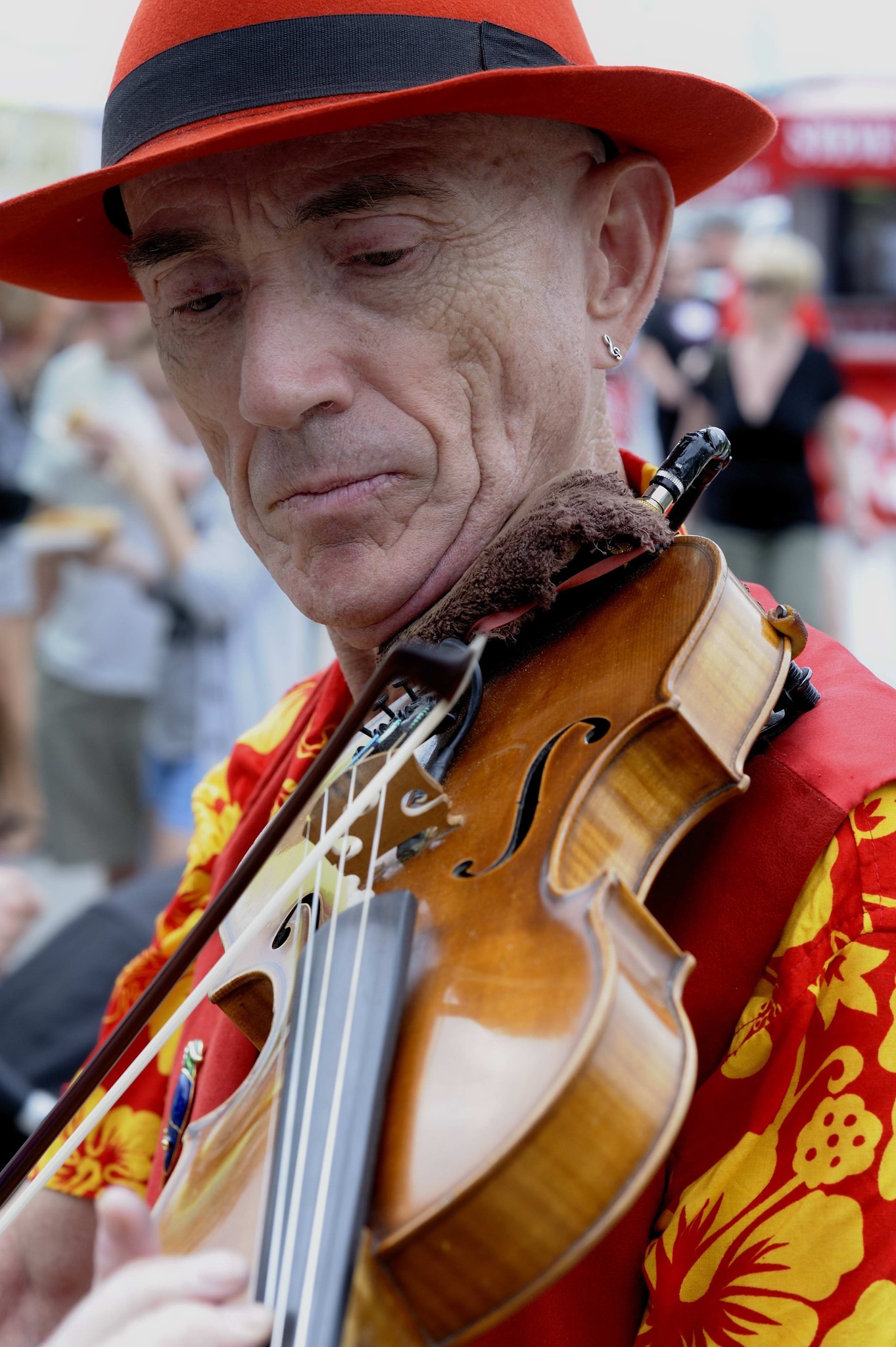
[154,534,795,1347]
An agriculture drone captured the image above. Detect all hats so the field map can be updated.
[0,0,777,304]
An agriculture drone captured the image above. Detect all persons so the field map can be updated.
[0,177,854,1163]
[1,0,895,1347]
